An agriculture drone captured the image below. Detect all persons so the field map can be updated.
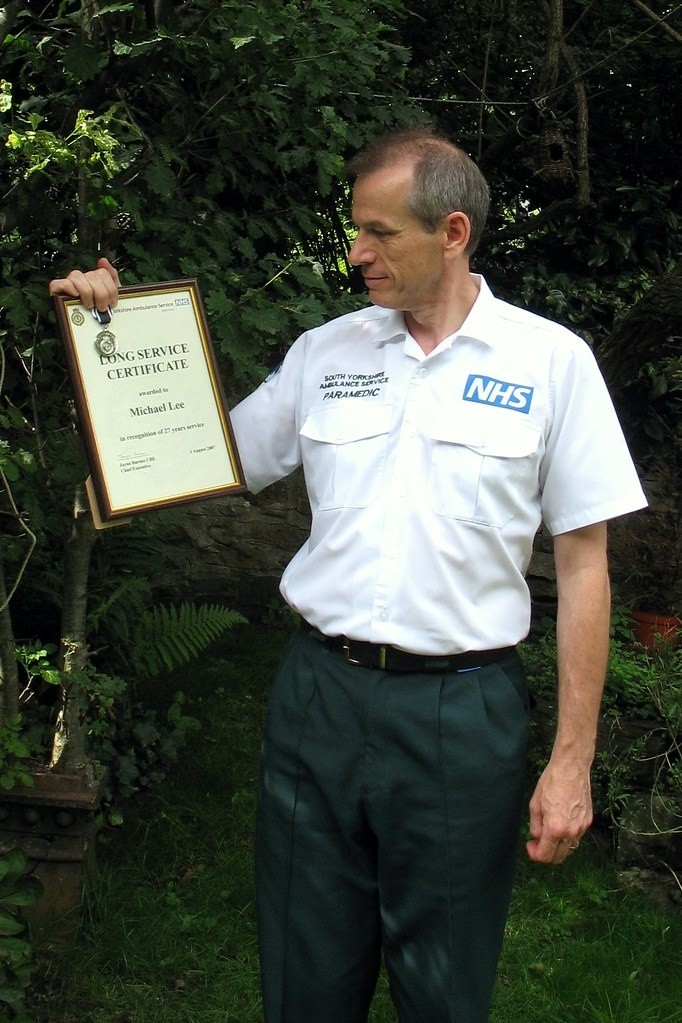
[49,126,650,1023]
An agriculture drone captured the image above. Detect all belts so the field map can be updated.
[300,617,501,671]
